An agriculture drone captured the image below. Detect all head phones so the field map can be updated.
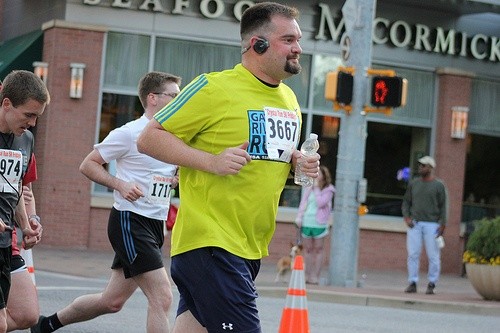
[241,40,268,54]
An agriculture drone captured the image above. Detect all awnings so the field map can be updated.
[0,30,44,83]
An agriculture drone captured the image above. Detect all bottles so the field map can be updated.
[435,233,445,248]
[294,134,320,189]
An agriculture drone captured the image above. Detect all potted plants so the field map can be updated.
[462,216,500,301]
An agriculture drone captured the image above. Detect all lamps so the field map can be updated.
[32,62,49,85]
[451,106,469,139]
[69,62,86,99]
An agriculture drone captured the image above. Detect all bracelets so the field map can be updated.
[29,215,40,223]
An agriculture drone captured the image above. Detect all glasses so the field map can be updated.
[146,91,177,99]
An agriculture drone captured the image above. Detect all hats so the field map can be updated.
[417,156,437,168]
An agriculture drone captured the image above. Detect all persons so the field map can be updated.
[297,166,333,285]
[31,72,181,333]
[402,156,448,294]
[137,3,321,333]
[0,70,51,333]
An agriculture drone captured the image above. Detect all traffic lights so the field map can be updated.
[324,71,354,104]
[371,76,408,107]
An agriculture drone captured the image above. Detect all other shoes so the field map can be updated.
[307,278,317,285]
[425,286,435,295]
[404,285,416,293]
[30,314,46,333]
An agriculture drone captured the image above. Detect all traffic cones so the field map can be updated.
[19,236,36,287]
[278,254,310,333]
